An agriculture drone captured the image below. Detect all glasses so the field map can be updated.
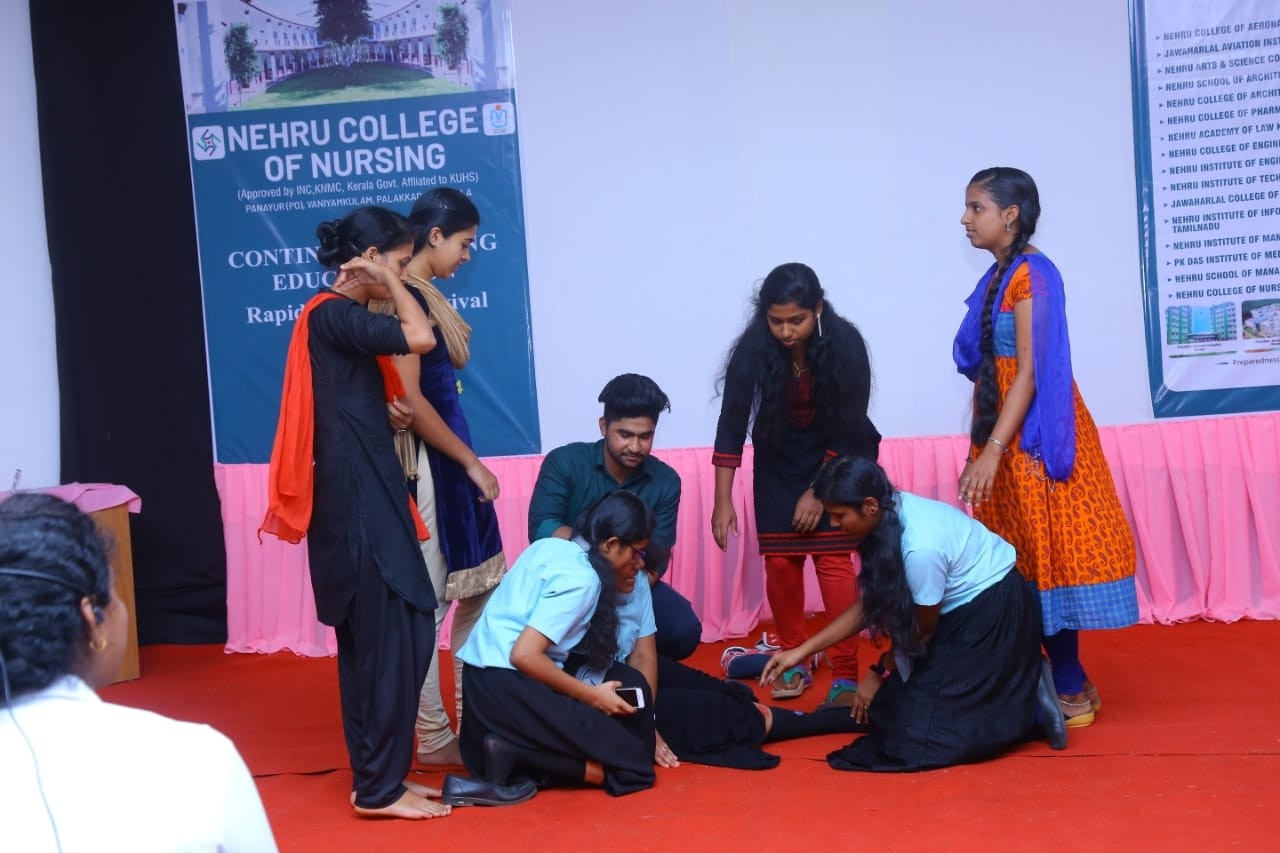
[629,543,646,560]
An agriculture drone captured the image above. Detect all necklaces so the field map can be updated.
[792,359,808,376]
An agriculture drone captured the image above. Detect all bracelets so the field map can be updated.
[868,648,891,679]
[987,437,1009,453]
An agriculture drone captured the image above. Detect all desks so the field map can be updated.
[0,481,142,691]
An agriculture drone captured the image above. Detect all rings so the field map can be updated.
[964,477,971,482]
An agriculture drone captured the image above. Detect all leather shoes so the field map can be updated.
[442,774,536,807]
[1035,653,1068,750]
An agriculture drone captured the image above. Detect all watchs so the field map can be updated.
[399,428,407,433]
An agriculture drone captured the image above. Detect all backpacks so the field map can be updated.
[721,632,835,678]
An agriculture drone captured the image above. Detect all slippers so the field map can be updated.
[1062,710,1095,728]
[772,668,810,698]
[829,680,855,705]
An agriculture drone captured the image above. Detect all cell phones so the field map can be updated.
[614,687,646,709]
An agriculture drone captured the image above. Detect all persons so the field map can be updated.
[711,262,882,707]
[952,168,1137,728]
[440,373,871,804]
[814,454,1067,774]
[370,188,509,766]
[260,205,450,820]
[1,490,278,853]
